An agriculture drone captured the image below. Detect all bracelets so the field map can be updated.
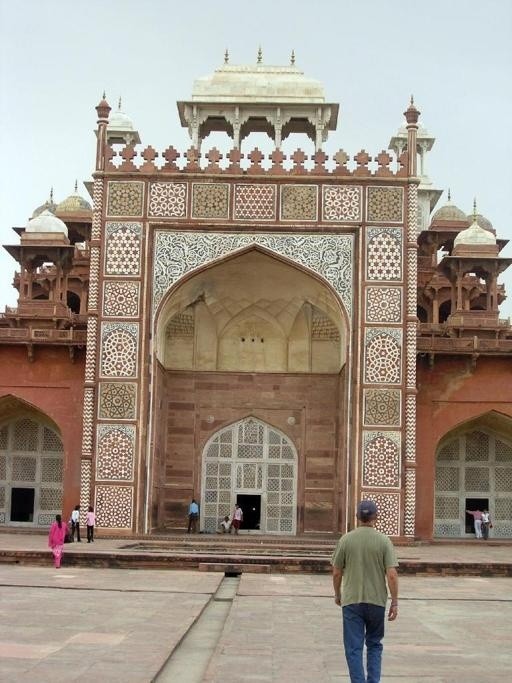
[390,603,398,607]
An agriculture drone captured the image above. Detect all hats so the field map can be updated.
[359,500,377,514]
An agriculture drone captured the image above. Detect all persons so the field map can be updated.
[465,508,483,539]
[84,505,97,543]
[480,508,492,539]
[186,498,199,533]
[68,504,82,542]
[229,502,244,534]
[49,513,69,566]
[330,499,400,683]
[216,514,234,533]
